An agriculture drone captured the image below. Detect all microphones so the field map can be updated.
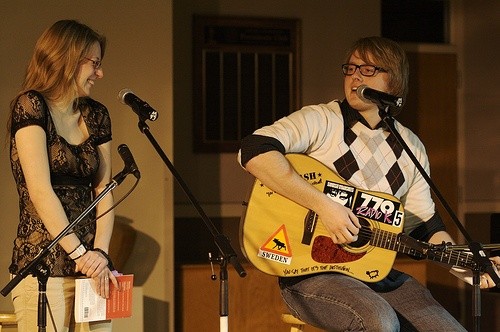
[357,85,402,108]
[118,144,141,179]
[117,88,158,122]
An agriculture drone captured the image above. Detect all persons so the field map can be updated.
[235,36,500,332]
[6,20,115,332]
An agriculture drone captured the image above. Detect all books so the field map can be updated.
[75,274,133,323]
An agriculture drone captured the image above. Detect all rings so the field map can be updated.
[105,278,109,280]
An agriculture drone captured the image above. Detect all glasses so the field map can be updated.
[82,56,102,69]
[342,64,387,76]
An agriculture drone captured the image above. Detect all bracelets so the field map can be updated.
[69,243,87,260]
[94,248,115,271]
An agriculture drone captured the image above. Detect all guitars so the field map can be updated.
[238,152,500,284]
